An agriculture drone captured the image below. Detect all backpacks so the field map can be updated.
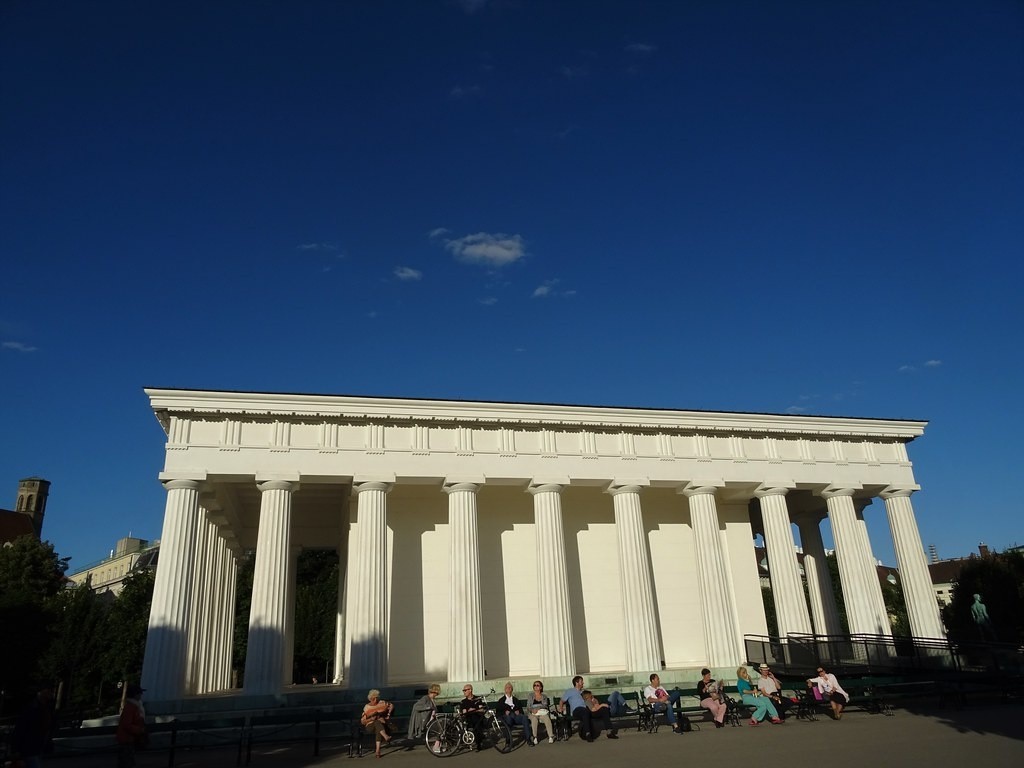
[679,714,700,732]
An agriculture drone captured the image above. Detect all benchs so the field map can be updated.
[437,664,1024,742]
[357,699,417,758]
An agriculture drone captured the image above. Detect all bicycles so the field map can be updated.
[424,687,513,758]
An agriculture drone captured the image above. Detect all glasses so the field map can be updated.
[817,670,824,673]
[533,684,542,687]
[430,691,439,696]
[462,688,470,691]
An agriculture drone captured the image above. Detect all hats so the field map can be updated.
[757,663,770,671]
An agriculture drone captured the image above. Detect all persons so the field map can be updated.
[496,682,535,750]
[559,676,637,741]
[361,689,394,759]
[806,666,850,720]
[643,674,683,733]
[970,594,997,642]
[116,684,147,768]
[459,684,493,752]
[737,667,785,726]
[408,684,447,753]
[757,664,792,721]
[698,669,727,728]
[527,681,554,744]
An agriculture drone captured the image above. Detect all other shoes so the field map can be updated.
[375,751,380,759]
[712,718,724,728]
[673,727,683,735]
[831,711,839,720]
[585,734,593,742]
[772,718,785,723]
[607,732,619,739]
[527,739,534,747]
[505,738,511,747]
[749,719,759,725]
[533,738,538,745]
[548,737,554,744]
[387,736,392,745]
[476,743,482,750]
[432,745,441,754]
[625,707,637,714]
[442,742,448,753]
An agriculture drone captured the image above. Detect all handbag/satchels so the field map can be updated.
[809,682,822,700]
[531,704,549,716]
